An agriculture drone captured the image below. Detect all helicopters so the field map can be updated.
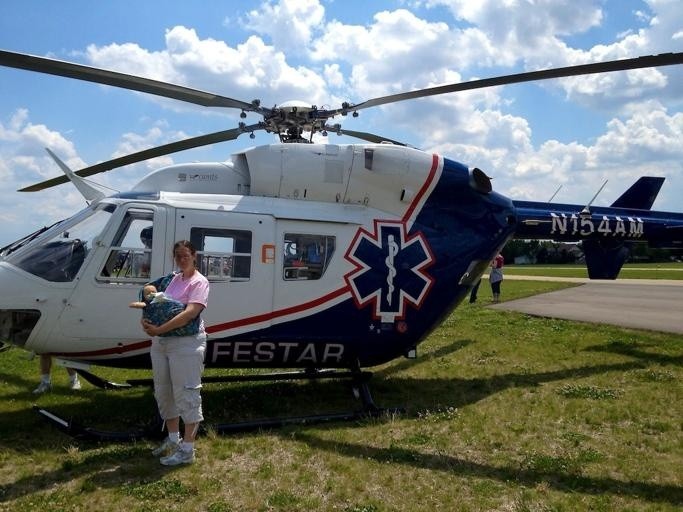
[0,50,682,445]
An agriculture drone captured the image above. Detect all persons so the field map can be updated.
[489,253,503,303]
[128,282,159,309]
[138,239,209,467]
[468,279,481,303]
[32,353,81,396]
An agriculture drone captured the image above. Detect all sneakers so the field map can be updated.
[31,381,51,394]
[69,380,81,390]
[152,438,178,457]
[160,449,196,465]
